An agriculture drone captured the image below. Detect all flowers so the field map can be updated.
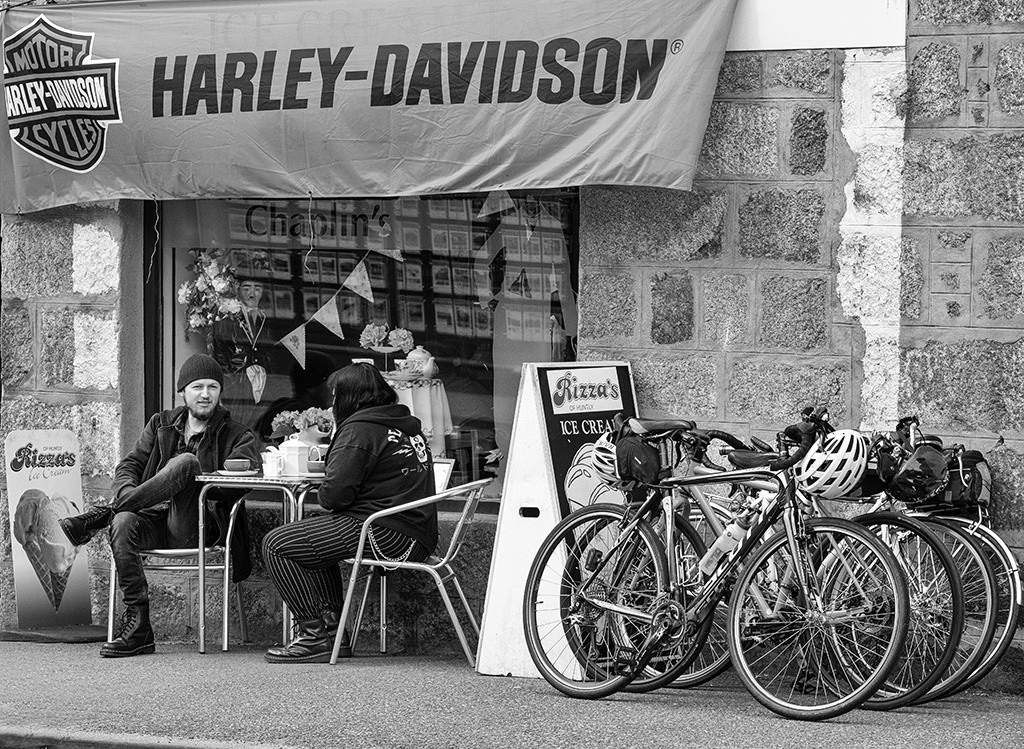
[359,321,414,354]
[269,406,333,439]
[177,249,254,357]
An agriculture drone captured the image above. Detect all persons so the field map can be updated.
[59,354,263,656]
[264,362,438,662]
[206,278,276,427]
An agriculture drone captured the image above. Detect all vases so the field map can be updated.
[285,424,332,460]
[370,346,402,372]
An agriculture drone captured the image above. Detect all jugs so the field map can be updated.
[260,452,284,476]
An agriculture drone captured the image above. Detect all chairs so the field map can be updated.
[330,477,495,668]
[107,490,249,653]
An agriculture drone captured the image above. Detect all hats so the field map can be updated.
[177,353,224,393]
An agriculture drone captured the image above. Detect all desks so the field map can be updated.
[193,476,324,654]
[379,370,453,459]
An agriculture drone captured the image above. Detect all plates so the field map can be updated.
[301,473,325,477]
[390,372,423,377]
[217,470,258,476]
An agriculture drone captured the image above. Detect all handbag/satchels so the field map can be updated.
[938,444,994,506]
[614,436,658,482]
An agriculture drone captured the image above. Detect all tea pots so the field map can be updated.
[407,345,439,379]
[265,434,321,476]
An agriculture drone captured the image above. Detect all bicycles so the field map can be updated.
[522,406,1022,723]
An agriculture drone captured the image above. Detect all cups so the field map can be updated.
[262,464,267,475]
[394,359,414,372]
[223,459,250,471]
[307,461,325,473]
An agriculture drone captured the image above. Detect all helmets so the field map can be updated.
[591,429,643,491]
[794,427,867,500]
[891,447,949,505]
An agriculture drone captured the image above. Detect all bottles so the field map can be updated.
[450,426,462,439]
[698,514,751,576]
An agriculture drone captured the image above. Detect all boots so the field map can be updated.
[313,609,354,658]
[264,615,334,665]
[59,506,116,547]
[100,602,156,656]
